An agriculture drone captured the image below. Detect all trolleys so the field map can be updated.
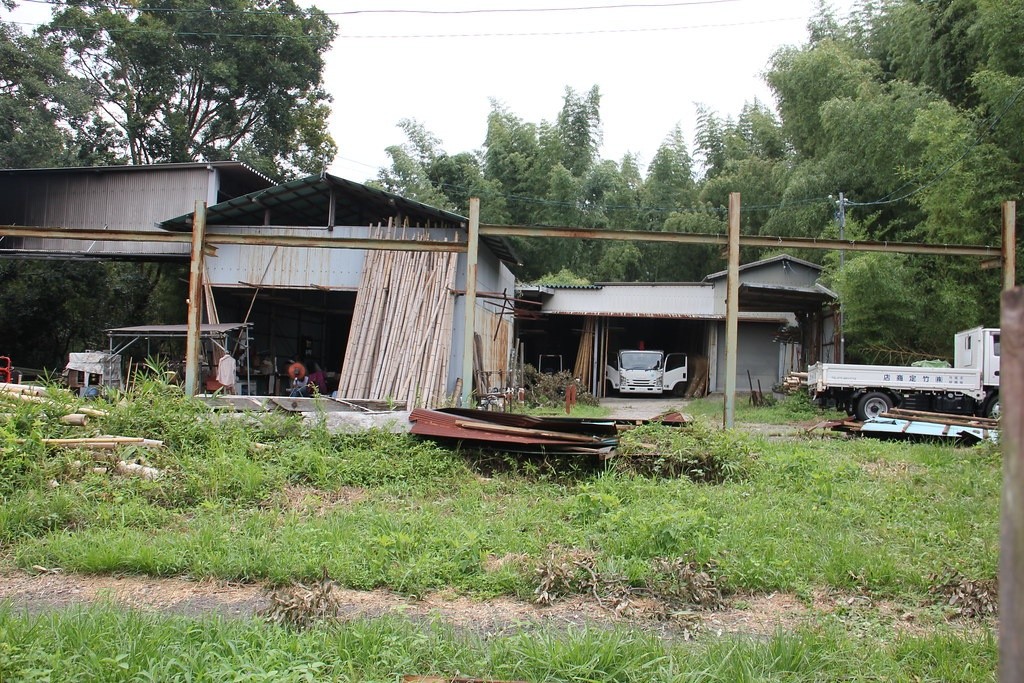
[747,370,769,407]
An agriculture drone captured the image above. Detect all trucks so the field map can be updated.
[807,325,1000,422]
[606,341,688,398]
